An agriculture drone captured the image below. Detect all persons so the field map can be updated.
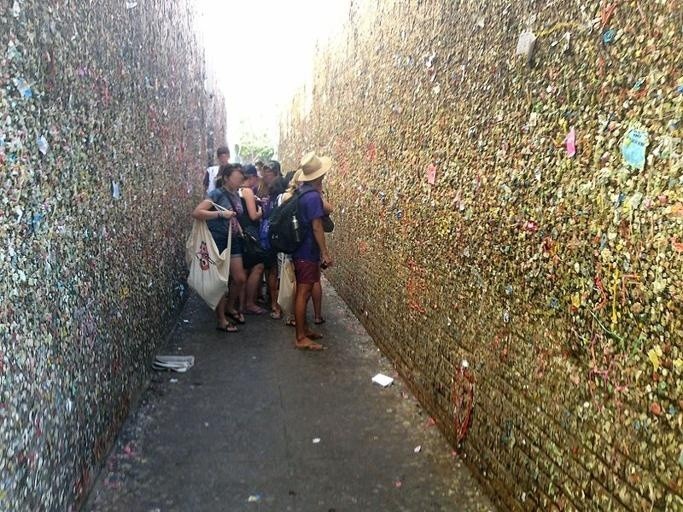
[189,146,334,352]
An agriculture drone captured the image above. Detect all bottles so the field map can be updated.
[290,214,302,243]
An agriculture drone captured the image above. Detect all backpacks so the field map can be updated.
[269,186,316,253]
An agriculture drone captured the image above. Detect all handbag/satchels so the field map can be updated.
[277,248,297,316]
[186,196,234,312]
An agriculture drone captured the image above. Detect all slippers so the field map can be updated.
[227,310,246,325]
[237,292,328,351]
[216,319,238,333]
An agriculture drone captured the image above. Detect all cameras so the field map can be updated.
[320,262,328,270]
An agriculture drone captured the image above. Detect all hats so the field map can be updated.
[296,150,333,182]
